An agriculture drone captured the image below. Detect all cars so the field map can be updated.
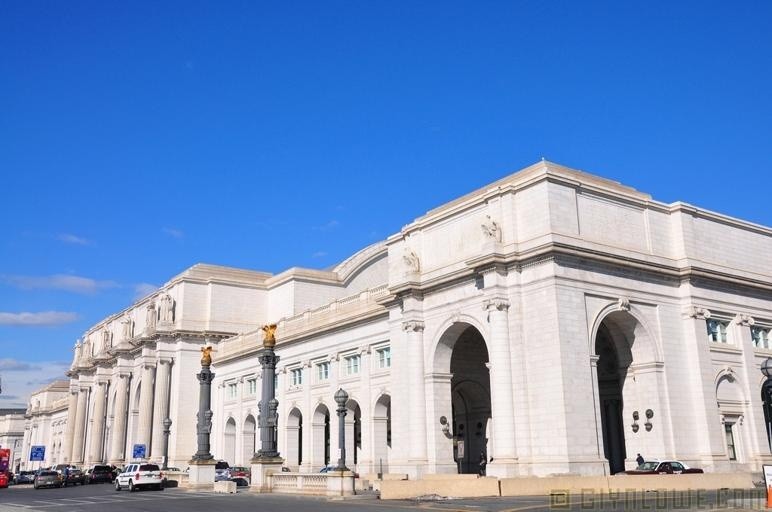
[615,460,703,474]
[319,468,359,478]
[0,464,113,489]
[182,461,250,486]
[115,463,165,491]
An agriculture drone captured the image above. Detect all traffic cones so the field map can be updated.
[767,485,772,509]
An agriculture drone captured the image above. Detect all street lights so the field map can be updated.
[162,418,172,467]
[193,346,215,460]
[761,361,772,441]
[257,326,280,456]
[334,388,348,468]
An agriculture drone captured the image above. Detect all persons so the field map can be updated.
[476,449,487,475]
[635,453,644,465]
